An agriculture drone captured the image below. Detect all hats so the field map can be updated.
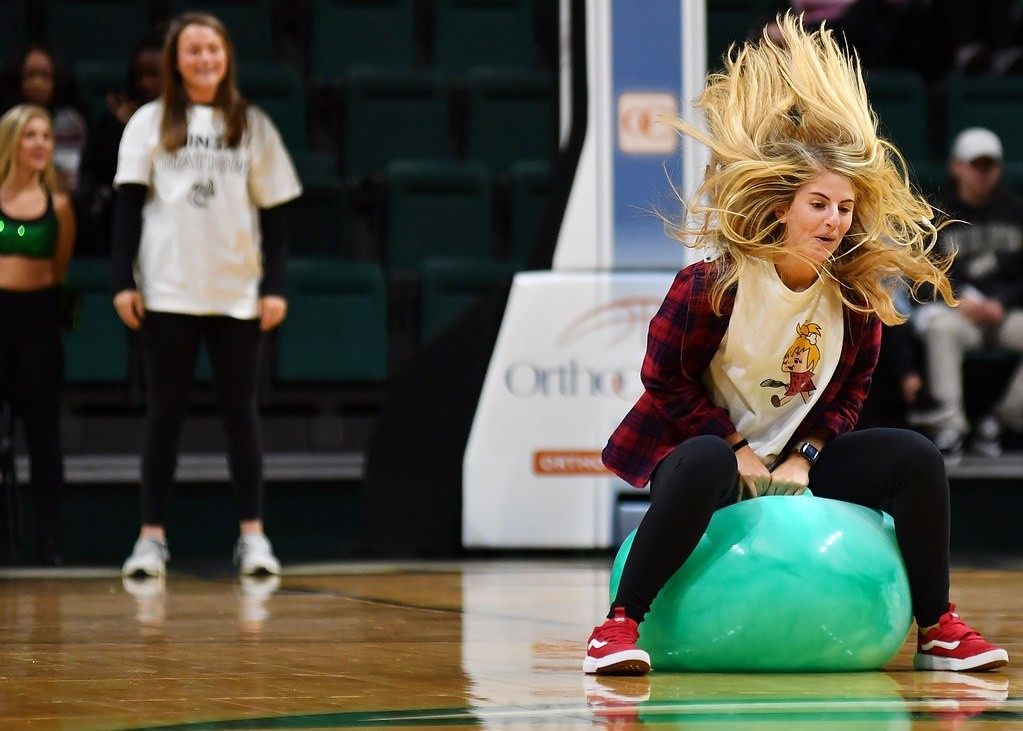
[956,129,1005,167]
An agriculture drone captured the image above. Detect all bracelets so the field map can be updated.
[732,439,748,452]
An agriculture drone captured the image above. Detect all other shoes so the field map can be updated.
[936,428,964,461]
[974,424,1002,455]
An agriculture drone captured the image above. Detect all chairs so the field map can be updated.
[0,0,1023,387]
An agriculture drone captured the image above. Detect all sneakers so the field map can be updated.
[913,603,1008,671]
[584,676,650,720]
[582,608,651,674]
[121,537,171,577]
[913,671,1008,714]
[235,534,280,574]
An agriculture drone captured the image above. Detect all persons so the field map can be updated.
[861,123,1023,465]
[0,11,300,579]
[584,14,1009,675]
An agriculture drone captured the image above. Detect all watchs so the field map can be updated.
[792,441,819,465]
